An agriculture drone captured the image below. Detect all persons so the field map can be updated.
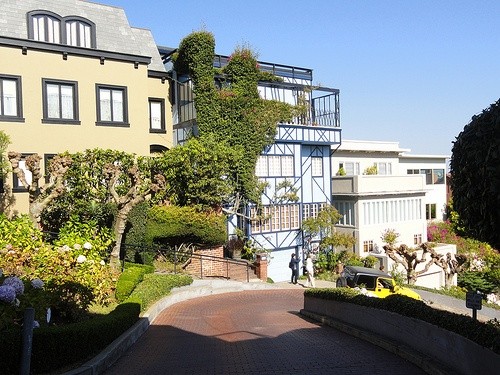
[379,266,385,272]
[305,253,316,288]
[289,252,301,284]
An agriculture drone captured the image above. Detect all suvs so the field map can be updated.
[336,265,420,301]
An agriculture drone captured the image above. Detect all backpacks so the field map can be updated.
[289,263,292,267]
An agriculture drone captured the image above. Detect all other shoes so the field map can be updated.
[292,282,299,285]
